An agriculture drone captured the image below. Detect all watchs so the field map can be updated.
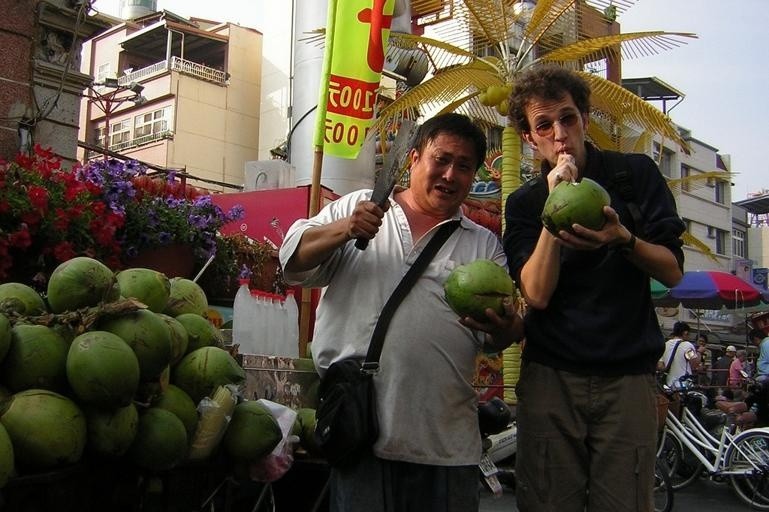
[615,234,637,257]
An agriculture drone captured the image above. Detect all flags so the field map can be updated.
[315,0,397,162]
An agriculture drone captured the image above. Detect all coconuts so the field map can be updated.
[541,177,610,240]
[1,256,320,490]
[441,258,517,323]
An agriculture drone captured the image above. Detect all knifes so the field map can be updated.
[353,119,419,254]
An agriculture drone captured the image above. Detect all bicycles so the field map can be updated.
[653,372,768,510]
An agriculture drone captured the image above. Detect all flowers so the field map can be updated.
[1,146,271,300]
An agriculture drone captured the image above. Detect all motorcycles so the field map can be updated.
[486,417,515,485]
[476,389,510,503]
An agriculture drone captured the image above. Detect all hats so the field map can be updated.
[727,346,736,352]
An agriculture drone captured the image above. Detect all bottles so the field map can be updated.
[230,276,301,361]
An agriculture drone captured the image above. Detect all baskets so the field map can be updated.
[658,394,680,432]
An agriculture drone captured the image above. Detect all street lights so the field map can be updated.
[85,69,145,163]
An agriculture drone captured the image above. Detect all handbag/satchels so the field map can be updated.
[315,357,381,459]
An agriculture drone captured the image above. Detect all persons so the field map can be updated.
[656,328,769,487]
[501,68,685,512]
[278,112,527,511]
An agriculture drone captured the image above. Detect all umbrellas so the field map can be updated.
[649,271,760,348]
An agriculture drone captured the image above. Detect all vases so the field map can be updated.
[226,241,279,298]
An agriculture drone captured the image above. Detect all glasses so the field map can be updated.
[531,111,579,138]
